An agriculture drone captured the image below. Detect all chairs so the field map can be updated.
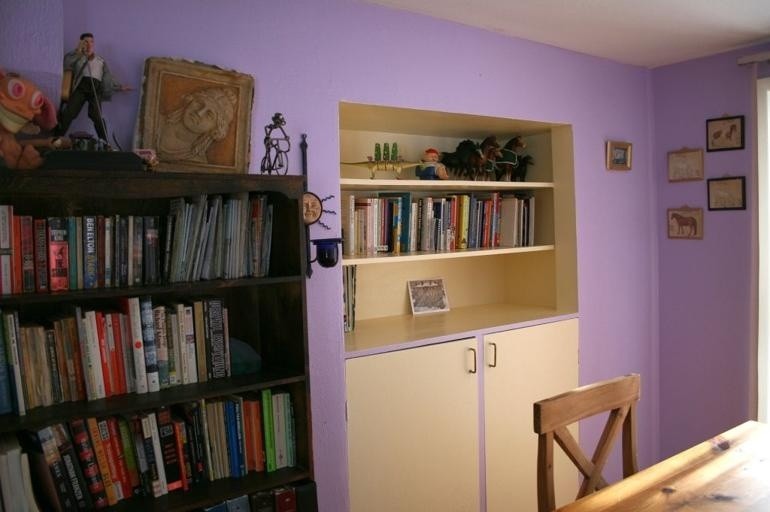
[533,374,641,512]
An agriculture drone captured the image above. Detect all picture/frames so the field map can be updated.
[606,140,633,171]
[667,149,702,183]
[140,56,254,172]
[706,115,744,152]
[408,278,450,315]
[667,206,703,239]
[707,177,746,211]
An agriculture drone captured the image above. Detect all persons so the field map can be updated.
[55,32,136,145]
[153,82,240,165]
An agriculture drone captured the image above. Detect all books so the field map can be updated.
[341,186,540,254]
[344,266,358,333]
[207,482,314,512]
[1,389,297,511]
[0,195,274,296]
[0,298,233,415]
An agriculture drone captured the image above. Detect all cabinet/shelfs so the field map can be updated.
[339,102,578,512]
[0,175,318,512]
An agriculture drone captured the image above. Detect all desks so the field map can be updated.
[550,419,770,512]
